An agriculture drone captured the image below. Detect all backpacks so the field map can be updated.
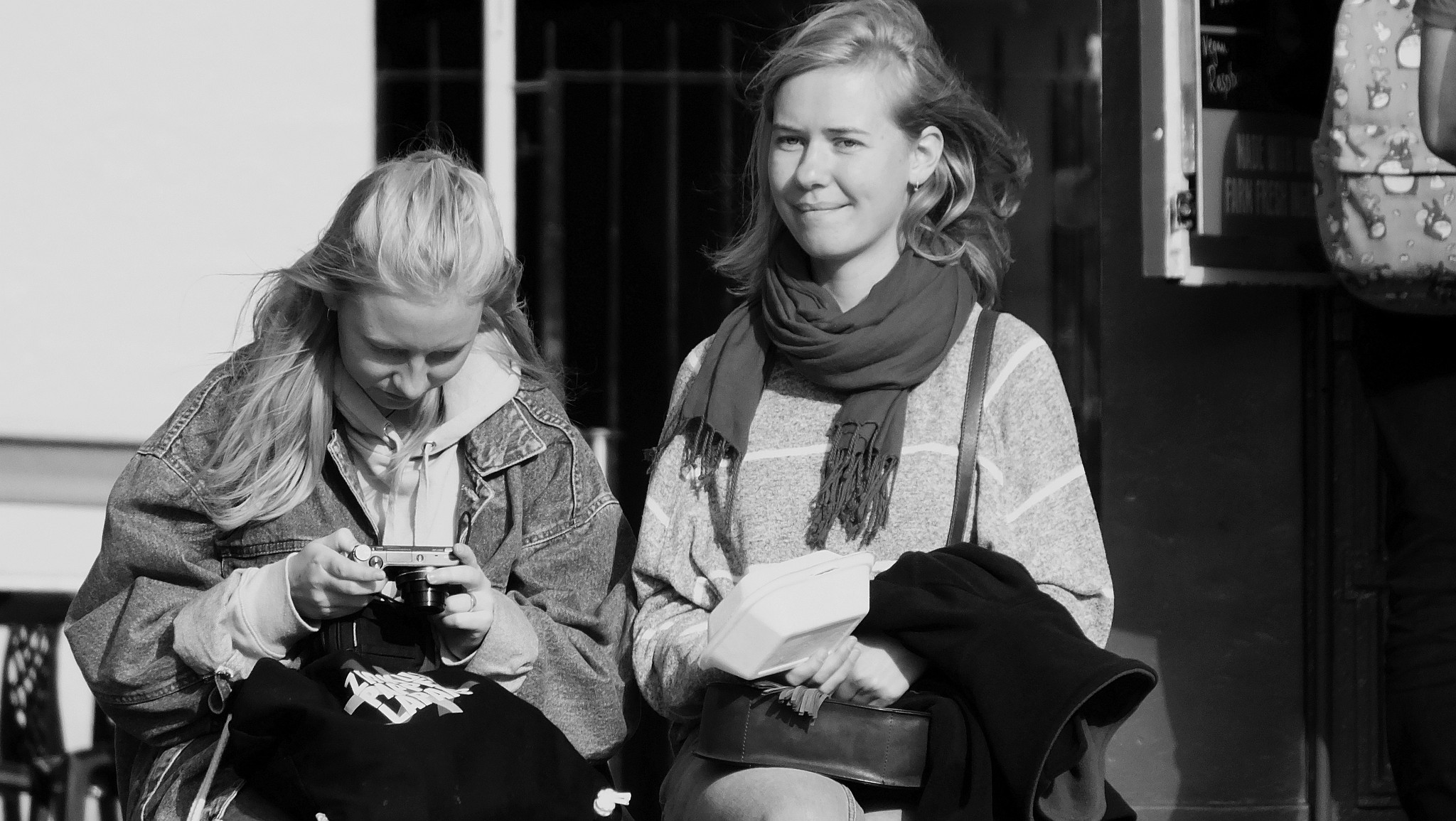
[1312,0,1455,316]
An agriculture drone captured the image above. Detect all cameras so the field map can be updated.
[339,544,461,616]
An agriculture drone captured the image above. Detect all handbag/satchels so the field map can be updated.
[692,680,933,788]
[228,655,622,821]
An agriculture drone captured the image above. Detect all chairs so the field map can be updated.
[0,589,114,821]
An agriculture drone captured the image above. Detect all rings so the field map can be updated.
[467,593,477,612]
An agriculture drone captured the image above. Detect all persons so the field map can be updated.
[64,146,640,821]
[632,0,1114,821]
